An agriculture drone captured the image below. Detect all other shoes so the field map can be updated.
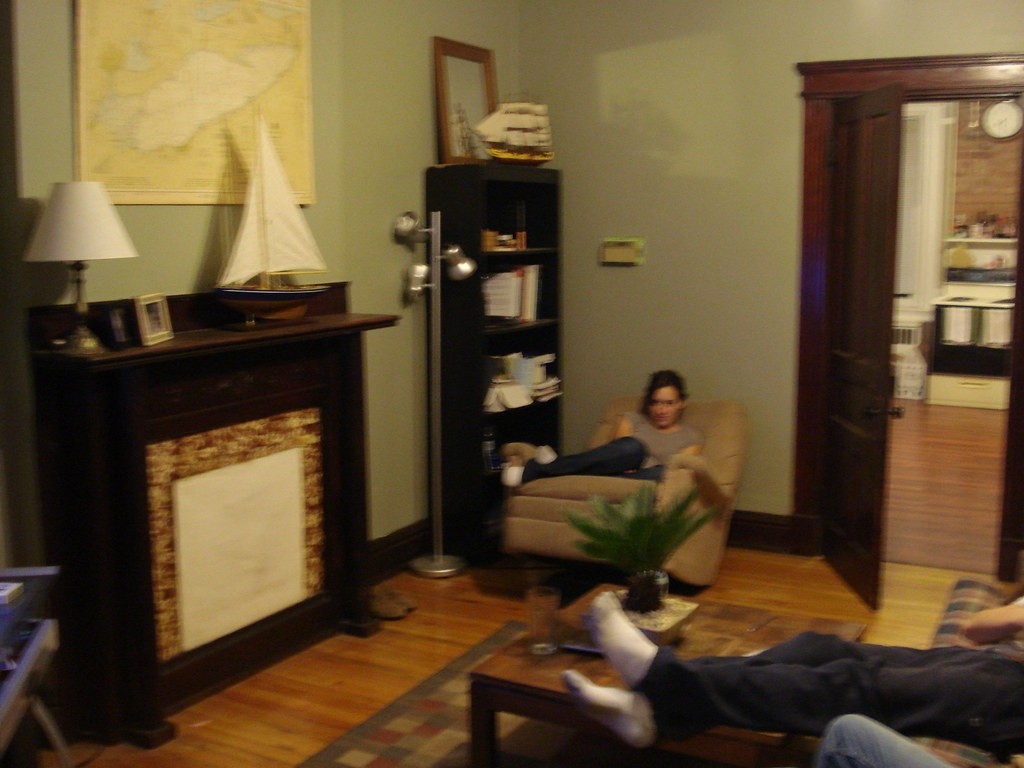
[377,584,418,609]
[369,599,403,620]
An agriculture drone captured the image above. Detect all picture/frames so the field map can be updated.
[434,35,499,168]
[101,292,177,350]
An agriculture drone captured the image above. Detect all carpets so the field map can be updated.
[295,618,821,768]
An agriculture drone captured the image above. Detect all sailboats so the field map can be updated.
[208,114,336,308]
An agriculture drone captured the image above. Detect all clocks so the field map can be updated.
[980,98,1024,142]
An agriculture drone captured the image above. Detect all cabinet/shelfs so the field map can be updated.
[927,232,1018,410]
[423,163,563,570]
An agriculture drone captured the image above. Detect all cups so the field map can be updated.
[526,586,559,655]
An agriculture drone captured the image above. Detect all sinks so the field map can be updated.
[976,296,1015,347]
[929,294,976,345]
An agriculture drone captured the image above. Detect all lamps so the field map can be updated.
[21,180,141,357]
[392,211,478,581]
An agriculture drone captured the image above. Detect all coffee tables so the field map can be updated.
[467,584,867,767]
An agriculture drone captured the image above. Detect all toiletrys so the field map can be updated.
[953,211,1017,238]
[950,245,971,267]
[985,255,1005,269]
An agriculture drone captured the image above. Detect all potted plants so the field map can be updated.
[568,478,727,645]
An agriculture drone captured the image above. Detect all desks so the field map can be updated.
[25,279,403,750]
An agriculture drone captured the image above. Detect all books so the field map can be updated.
[480,264,540,322]
[482,351,563,413]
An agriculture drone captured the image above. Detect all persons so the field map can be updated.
[501,369,705,488]
[815,712,958,768]
[560,589,1024,755]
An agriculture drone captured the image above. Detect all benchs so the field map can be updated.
[913,574,1020,767]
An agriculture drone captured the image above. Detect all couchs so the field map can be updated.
[502,397,751,588]
